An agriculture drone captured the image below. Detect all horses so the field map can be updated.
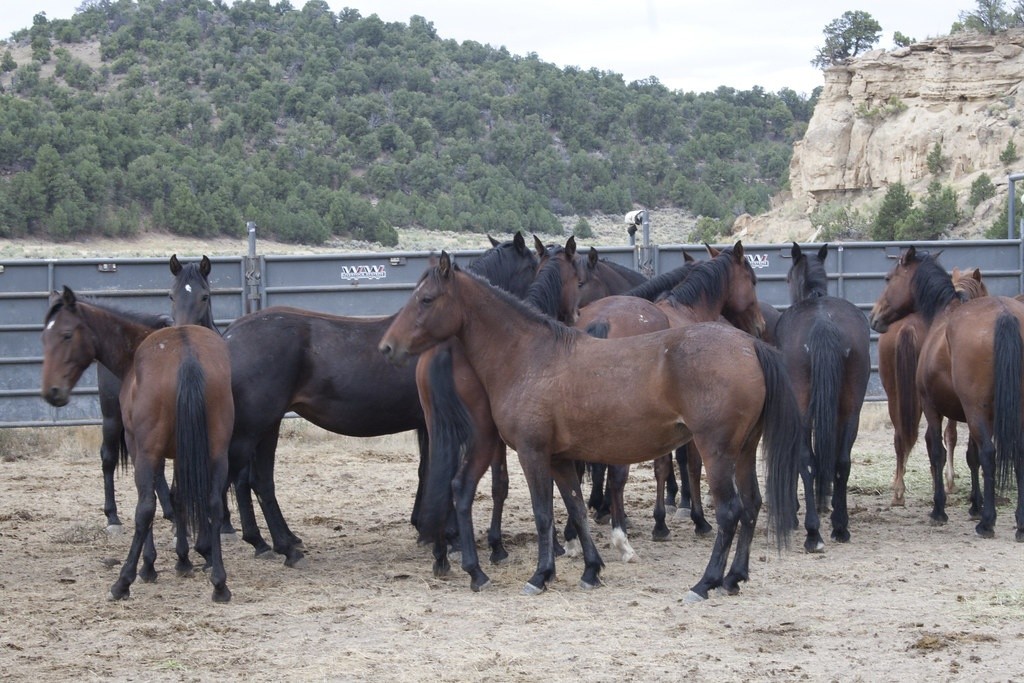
[411,230,1024,595]
[219,230,536,571]
[95,253,240,534]
[40,281,239,604]
[376,248,793,602]
